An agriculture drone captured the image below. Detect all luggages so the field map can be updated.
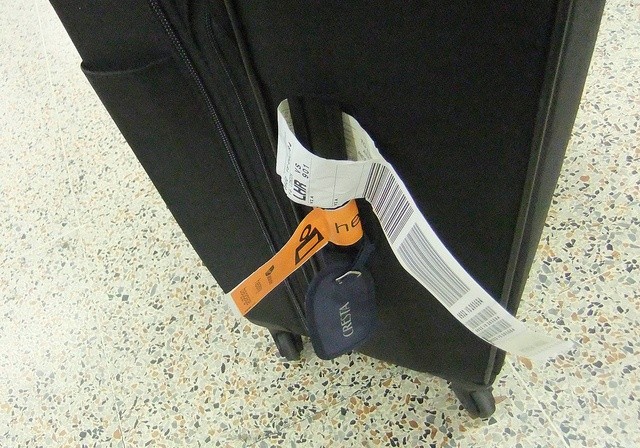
[48,0,607,419]
